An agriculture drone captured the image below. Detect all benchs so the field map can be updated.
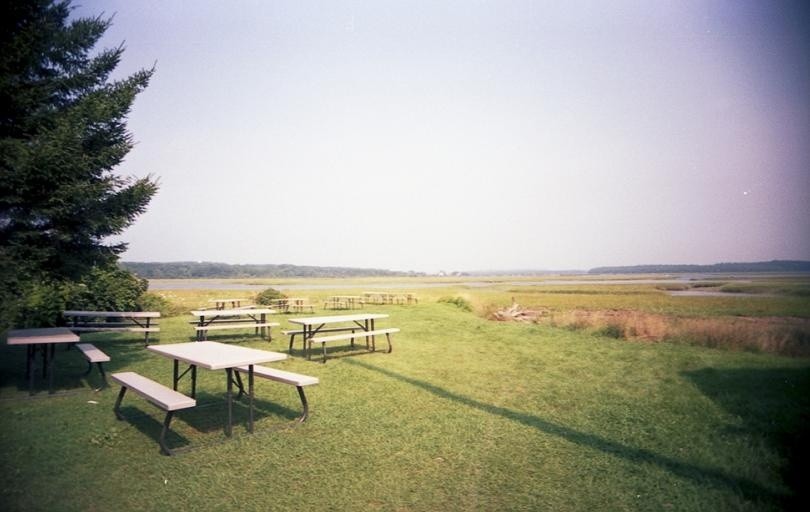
[66,321,158,326]
[110,371,197,456]
[189,293,418,364]
[74,343,111,394]
[66,326,161,351]
[233,364,318,433]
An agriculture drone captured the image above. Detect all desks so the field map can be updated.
[6,326,80,396]
[146,340,288,439]
[63,310,160,346]
